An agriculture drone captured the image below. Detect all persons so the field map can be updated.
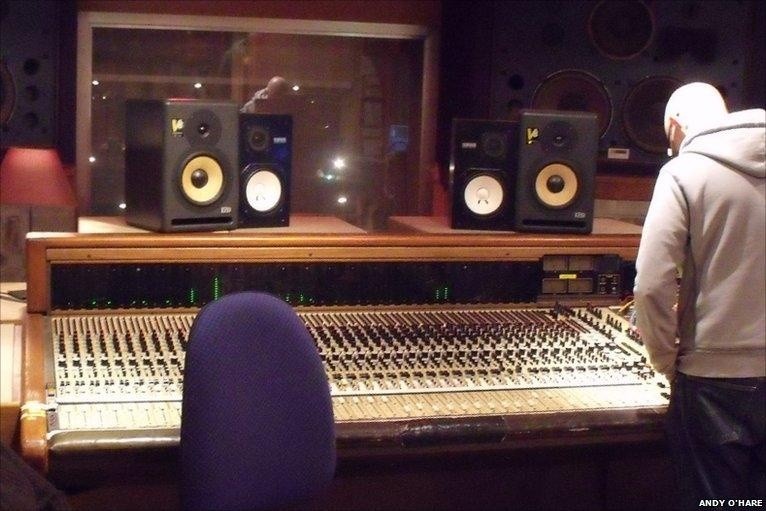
[236,75,289,118]
[632,80,766,510]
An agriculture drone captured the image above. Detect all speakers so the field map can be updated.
[517,109,599,234]
[124,97,239,231]
[487,1,755,169]
[449,117,518,231]
[239,112,292,228]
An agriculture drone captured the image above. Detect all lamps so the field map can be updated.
[0,147,80,300]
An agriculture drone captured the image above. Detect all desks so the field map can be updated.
[16,216,666,477]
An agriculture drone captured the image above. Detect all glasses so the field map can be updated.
[667,113,681,157]
